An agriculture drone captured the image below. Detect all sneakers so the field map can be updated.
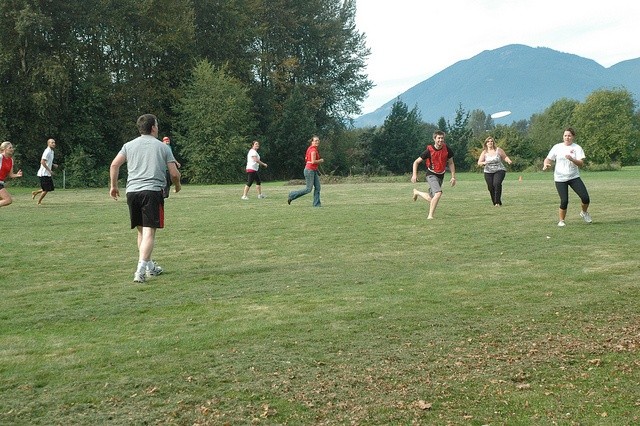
[558,221,566,227]
[580,212,593,223]
[37,202,42,205]
[258,194,267,199]
[495,204,501,207]
[288,195,292,205]
[133,272,149,283]
[241,196,249,200]
[146,266,163,276]
[32,191,36,199]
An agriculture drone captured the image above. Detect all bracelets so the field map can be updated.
[451,177,456,181]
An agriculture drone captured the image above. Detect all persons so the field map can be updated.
[32,139,58,205]
[0,141,23,206]
[478,136,512,207]
[542,129,592,226]
[241,140,268,200]
[109,114,182,283]
[287,135,324,207]
[161,137,181,198]
[411,130,455,219]
